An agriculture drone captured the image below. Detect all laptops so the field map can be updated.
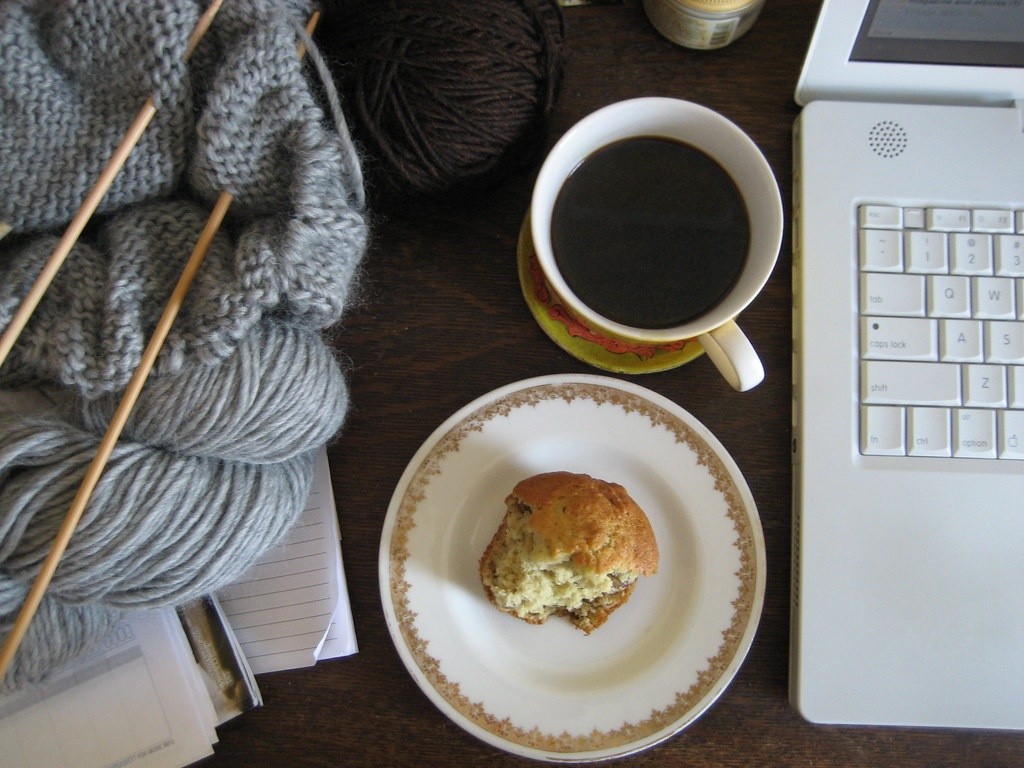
[791,0,1024,735]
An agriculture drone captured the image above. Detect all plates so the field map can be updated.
[379,375,765,763]
[518,207,739,374]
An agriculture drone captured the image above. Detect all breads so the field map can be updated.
[477,471,660,635]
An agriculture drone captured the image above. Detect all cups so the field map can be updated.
[530,97,783,392]
